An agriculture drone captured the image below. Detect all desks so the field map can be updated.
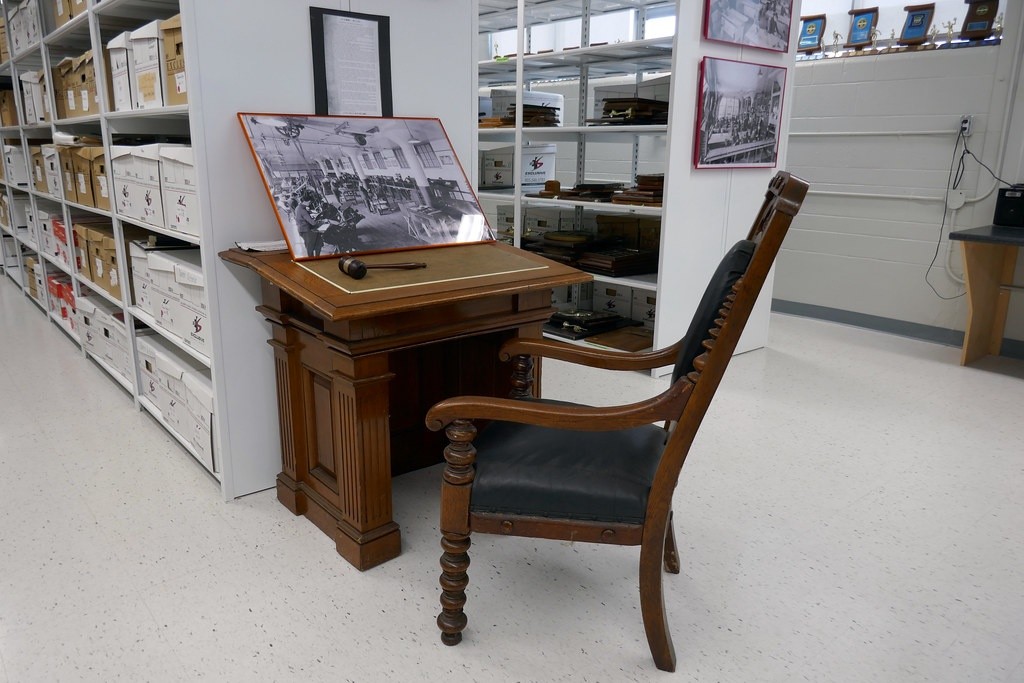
[217,242,595,573]
[947,227,1024,367]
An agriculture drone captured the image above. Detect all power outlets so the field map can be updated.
[960,115,974,136]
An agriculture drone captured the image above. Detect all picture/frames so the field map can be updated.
[694,56,788,170]
[238,110,497,260]
[703,0,794,54]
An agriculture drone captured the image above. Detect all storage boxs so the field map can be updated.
[594,281,657,326]
[479,142,557,187]
[497,203,597,239]
[479,86,564,127]
[0,0,220,482]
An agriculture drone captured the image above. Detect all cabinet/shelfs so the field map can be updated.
[475,0,802,380]
[0,0,480,503]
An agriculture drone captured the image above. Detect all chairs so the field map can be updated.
[425,170,811,672]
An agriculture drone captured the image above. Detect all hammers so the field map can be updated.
[339,255,428,279]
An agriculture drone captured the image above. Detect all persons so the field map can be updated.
[330,177,343,205]
[277,176,299,215]
[358,181,373,213]
[294,196,324,257]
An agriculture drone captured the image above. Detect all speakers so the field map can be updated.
[994,188,1024,228]
